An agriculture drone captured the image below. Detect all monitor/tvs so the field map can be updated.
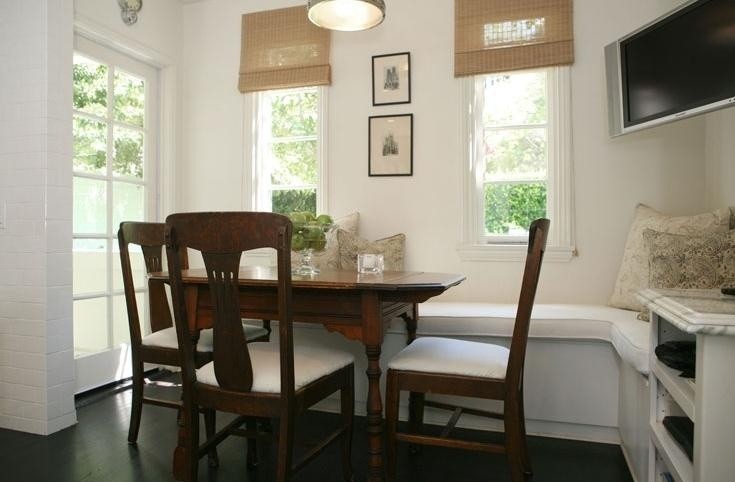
[604,0,735,140]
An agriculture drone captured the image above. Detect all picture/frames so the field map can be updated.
[369,114,412,176]
[372,52,411,105]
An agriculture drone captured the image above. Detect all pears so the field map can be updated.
[308,220,318,227]
[293,225,305,236]
[290,233,305,252]
[289,211,306,226]
[309,233,325,251]
[302,210,315,222]
[317,214,332,232]
[303,227,321,240]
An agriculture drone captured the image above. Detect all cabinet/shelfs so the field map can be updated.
[639,289,735,482]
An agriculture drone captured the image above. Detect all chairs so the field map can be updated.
[118,221,271,468]
[165,212,355,482]
[385,219,550,482]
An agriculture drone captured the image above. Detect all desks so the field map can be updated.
[145,266,467,482]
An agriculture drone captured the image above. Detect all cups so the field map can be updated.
[357,253,384,274]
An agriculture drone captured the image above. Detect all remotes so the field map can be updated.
[721,287,735,295]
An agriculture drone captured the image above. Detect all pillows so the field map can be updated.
[607,203,734,312]
[337,229,406,272]
[637,229,735,321]
[290,210,360,270]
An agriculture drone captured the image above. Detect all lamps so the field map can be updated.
[308,0,386,32]
